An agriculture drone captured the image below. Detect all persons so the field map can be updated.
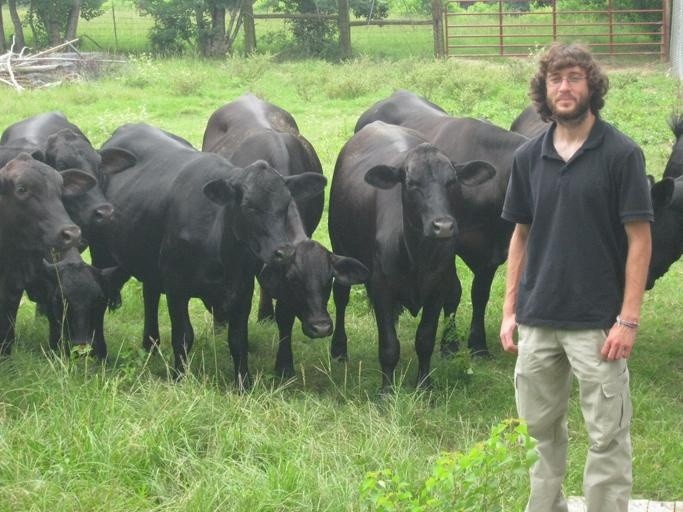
[500,44,655,512]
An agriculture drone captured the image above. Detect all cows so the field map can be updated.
[0,88,681,399]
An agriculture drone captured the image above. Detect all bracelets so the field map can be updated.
[616,316,641,329]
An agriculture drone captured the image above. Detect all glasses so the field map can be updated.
[544,72,587,87]
